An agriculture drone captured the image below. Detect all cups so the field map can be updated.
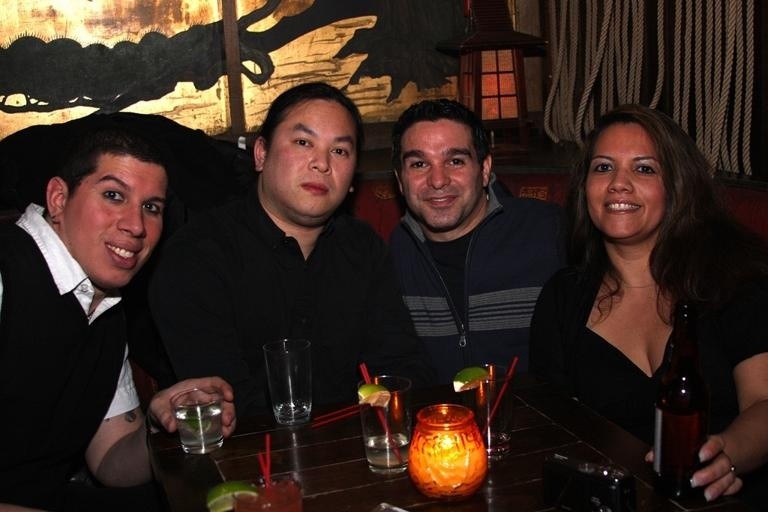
[262,337,314,425]
[469,363,512,457]
[169,386,225,456]
[357,375,413,472]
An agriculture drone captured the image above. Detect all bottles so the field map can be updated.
[651,301,714,495]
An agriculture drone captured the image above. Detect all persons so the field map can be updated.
[1,114,236,512]
[153,80,390,429]
[385,97,582,382]
[529,102,768,512]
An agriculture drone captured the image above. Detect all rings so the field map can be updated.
[731,465,736,472]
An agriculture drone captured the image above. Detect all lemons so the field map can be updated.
[356,383,390,408]
[454,365,489,393]
[204,481,261,510]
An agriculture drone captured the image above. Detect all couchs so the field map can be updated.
[345,163,766,277]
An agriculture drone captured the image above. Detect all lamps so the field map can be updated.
[436,1,548,166]
